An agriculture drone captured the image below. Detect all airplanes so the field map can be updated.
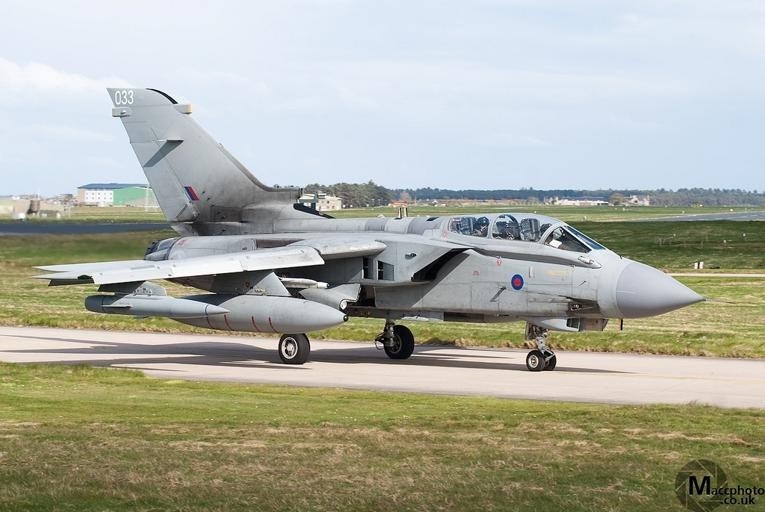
[30,86,707,372]
